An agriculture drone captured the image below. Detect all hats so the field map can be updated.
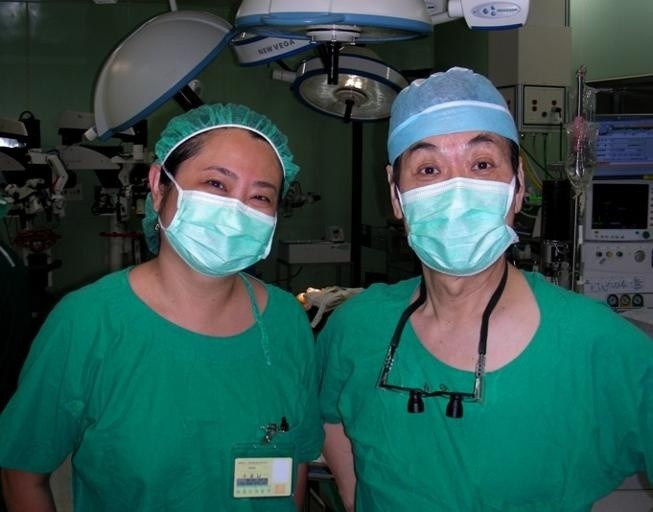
[141,103,299,253]
[387,67,520,165]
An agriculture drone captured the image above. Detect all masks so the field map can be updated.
[396,174,520,276]
[156,164,278,278]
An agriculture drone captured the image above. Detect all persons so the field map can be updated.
[316,64,650,512]
[0,100,324,512]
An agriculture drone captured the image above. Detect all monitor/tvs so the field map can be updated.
[583,179,653,241]
